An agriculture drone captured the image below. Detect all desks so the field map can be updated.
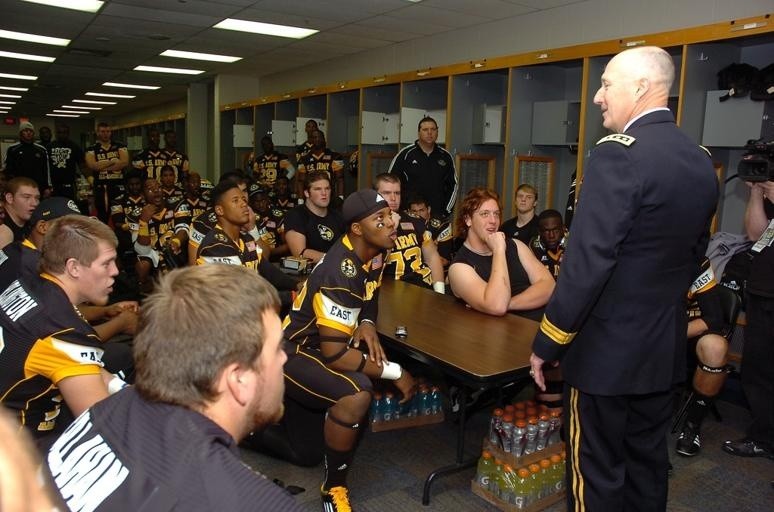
[274,263,542,507]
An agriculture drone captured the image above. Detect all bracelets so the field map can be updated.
[380,360,403,380]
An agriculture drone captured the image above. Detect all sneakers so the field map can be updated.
[723,439,769,457]
[675,426,701,456]
[321,481,353,512]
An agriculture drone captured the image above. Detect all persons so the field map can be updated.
[723,179,773,462]
[0,214,131,453]
[1,198,139,381]
[369,173,446,294]
[388,115,459,219]
[38,264,307,512]
[448,183,569,322]
[530,46,719,511]
[280,189,415,512]
[1,119,346,279]
[676,257,730,455]
[0,406,56,511]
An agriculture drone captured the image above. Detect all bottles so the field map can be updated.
[370,384,445,425]
[473,396,567,508]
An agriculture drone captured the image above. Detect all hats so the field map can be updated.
[343,188,389,225]
[19,121,34,132]
[31,196,99,225]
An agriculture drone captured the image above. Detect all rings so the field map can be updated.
[529,369,535,377]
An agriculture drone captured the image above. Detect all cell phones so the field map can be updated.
[395,324,407,338]
